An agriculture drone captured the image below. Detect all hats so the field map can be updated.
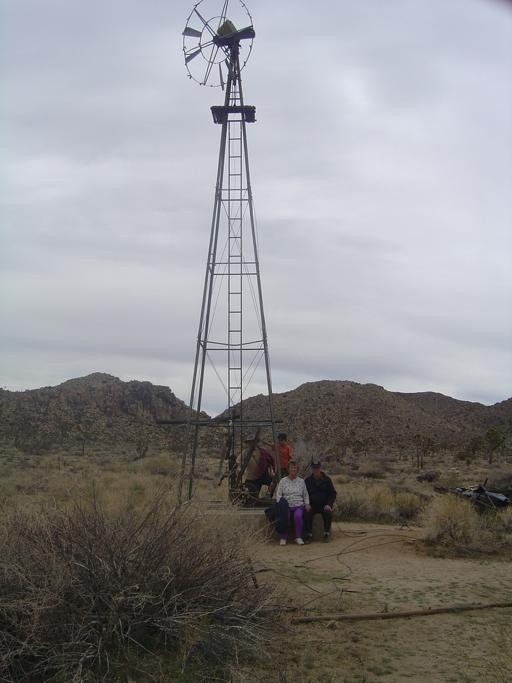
[310,460,322,468]
[243,434,260,444]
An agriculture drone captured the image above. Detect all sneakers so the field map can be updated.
[322,533,329,542]
[304,535,313,544]
[279,538,287,545]
[293,537,305,545]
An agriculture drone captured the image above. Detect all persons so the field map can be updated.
[276,460,311,546]
[263,432,293,478]
[304,461,336,542]
[231,434,278,500]
